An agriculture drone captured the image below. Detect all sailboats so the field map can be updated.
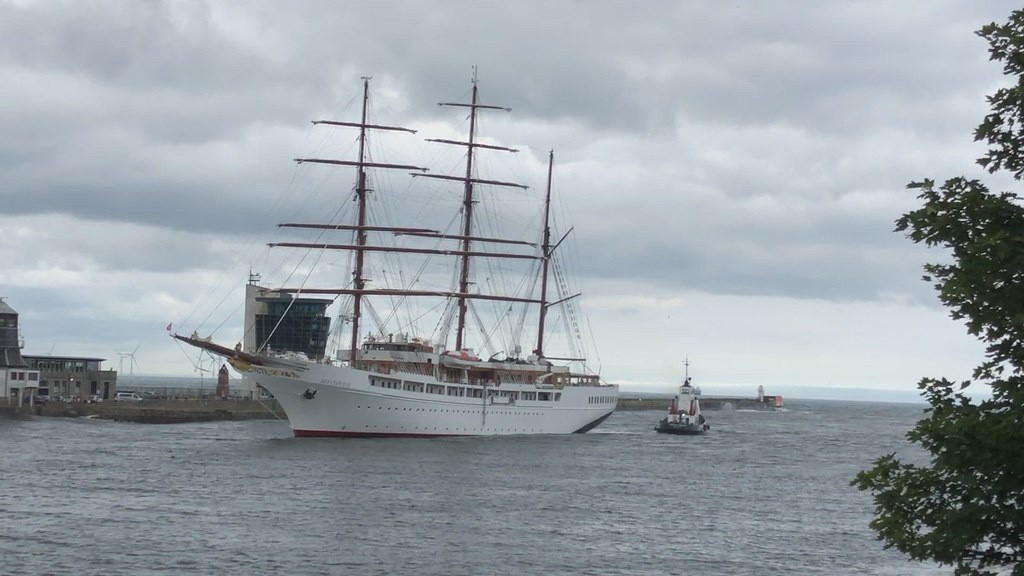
[167,61,620,438]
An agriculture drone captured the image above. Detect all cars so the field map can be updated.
[24,393,80,404]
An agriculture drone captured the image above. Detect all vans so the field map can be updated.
[114,391,144,402]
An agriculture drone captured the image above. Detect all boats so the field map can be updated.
[653,353,710,434]
[439,347,484,371]
